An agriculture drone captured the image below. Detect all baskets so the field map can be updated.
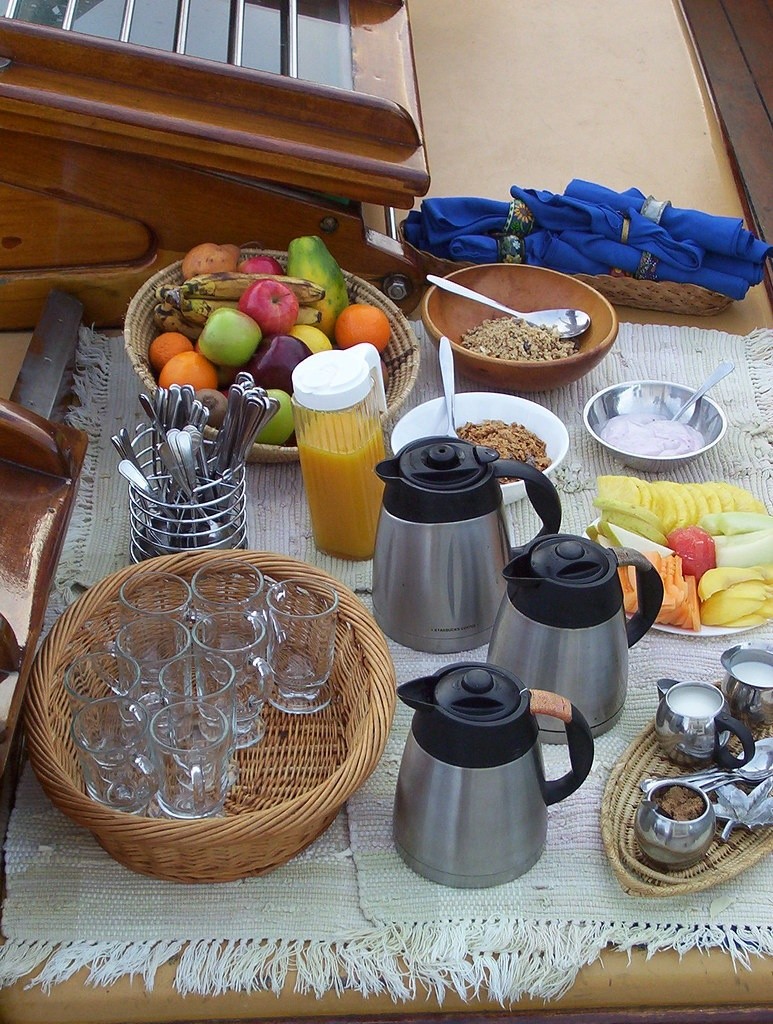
[399,220,735,313]
[123,248,420,463]
[603,680,773,897]
[24,548,396,884]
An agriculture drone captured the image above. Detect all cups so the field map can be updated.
[64,560,337,819]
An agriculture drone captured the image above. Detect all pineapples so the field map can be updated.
[591,474,768,535]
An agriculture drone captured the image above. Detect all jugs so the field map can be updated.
[290,343,389,561]
[390,661,596,890]
[369,434,562,654]
[485,535,663,741]
[655,679,754,771]
[719,643,773,729]
[633,780,716,872]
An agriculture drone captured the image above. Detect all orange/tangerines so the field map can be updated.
[148,332,218,393]
[334,304,390,353]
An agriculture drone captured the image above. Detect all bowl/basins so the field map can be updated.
[390,390,568,506]
[422,264,619,390]
[583,381,726,472]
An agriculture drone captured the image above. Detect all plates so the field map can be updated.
[583,516,771,634]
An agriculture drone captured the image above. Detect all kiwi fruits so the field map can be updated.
[195,389,229,427]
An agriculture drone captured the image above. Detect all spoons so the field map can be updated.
[439,335,460,439]
[426,273,590,337]
[641,738,773,796]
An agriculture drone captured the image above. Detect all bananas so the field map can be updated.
[154,271,326,339]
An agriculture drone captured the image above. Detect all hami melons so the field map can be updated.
[610,551,702,632]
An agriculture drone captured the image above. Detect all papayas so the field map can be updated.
[288,236,349,346]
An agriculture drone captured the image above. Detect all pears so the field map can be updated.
[587,499,676,560]
[182,243,241,279]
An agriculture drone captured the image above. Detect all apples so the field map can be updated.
[196,280,390,445]
[237,256,285,275]
